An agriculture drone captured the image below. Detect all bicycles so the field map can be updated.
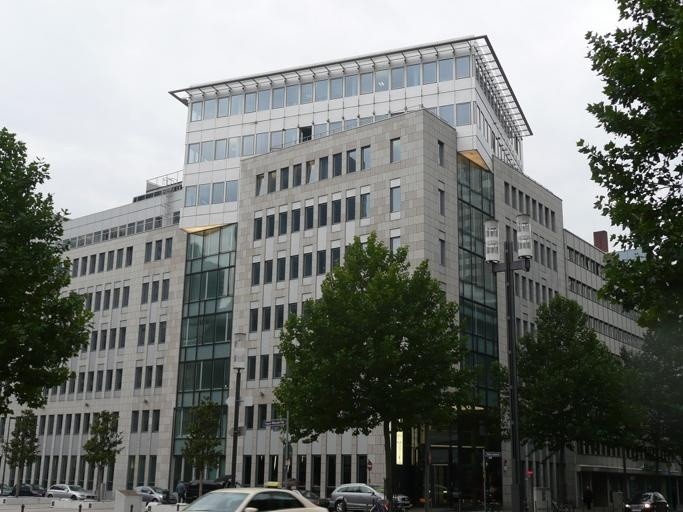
[552,495,570,512]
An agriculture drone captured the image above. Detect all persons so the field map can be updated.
[222,479,231,489]
[583,484,593,510]
[185,482,193,504]
[175,480,186,503]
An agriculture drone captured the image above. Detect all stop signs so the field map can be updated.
[527,468,533,476]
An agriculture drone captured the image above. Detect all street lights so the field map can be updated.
[482,213,534,511]
[229,332,248,487]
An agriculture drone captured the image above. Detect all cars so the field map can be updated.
[624,491,669,512]
[0,481,98,500]
[424,484,448,499]
[134,486,177,504]
[330,482,414,511]
[177,480,331,512]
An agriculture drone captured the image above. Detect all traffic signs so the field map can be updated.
[262,419,287,426]
